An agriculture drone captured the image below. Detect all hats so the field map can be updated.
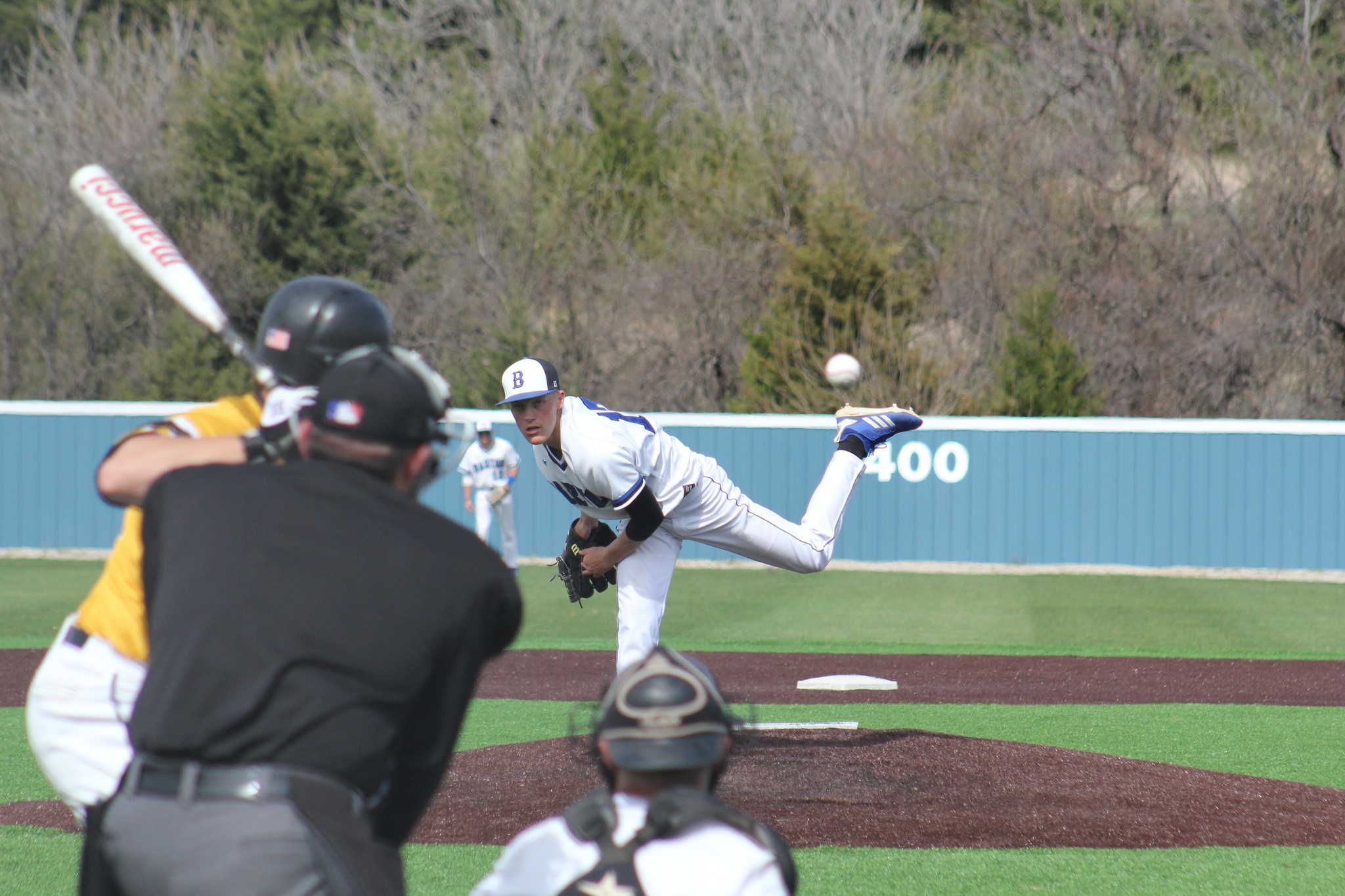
[474,418,493,434]
[296,352,450,451]
[494,357,561,406]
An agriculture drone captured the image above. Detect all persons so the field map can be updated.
[463,416,522,574]
[25,276,392,831]
[494,356,923,677]
[78,342,523,896]
[469,644,796,896]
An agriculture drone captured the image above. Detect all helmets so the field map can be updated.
[601,654,728,773]
[252,275,392,387]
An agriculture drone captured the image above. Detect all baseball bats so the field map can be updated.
[68,163,279,391]
[485,480,511,541]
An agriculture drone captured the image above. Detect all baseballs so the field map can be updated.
[824,353,861,391]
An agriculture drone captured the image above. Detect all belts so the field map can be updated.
[121,763,293,805]
[65,627,88,646]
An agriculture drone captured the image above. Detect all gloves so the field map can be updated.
[239,386,318,464]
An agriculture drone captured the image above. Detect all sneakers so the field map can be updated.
[834,402,923,457]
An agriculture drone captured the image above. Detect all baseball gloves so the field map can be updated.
[487,486,507,505]
[558,517,618,603]
